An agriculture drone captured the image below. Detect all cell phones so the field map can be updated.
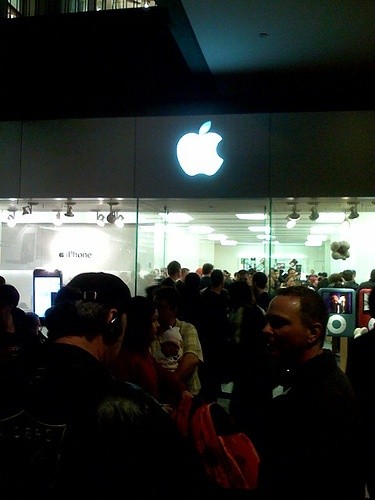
[33,268,62,326]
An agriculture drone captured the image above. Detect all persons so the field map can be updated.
[0,261,375,500]
[331,294,343,313]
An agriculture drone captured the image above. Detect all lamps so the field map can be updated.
[160,212,279,247]
[286,198,360,247]
[7,200,127,229]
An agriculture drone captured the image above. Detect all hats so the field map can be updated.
[45,273,132,332]
[160,326,183,348]
[168,263,179,272]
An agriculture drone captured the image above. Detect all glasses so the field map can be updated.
[156,303,171,309]
[265,313,292,329]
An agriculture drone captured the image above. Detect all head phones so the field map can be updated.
[102,311,123,347]
